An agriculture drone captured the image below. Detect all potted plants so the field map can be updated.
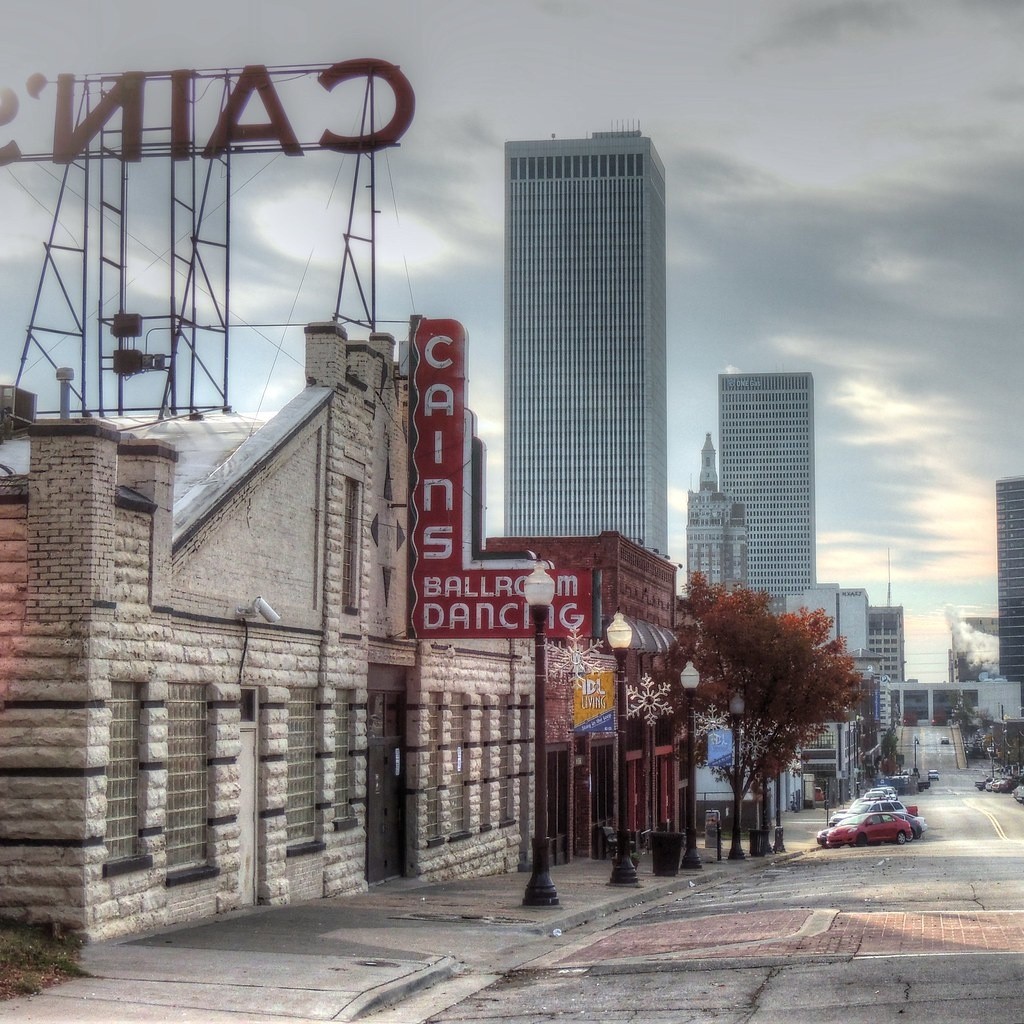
[612,854,639,870]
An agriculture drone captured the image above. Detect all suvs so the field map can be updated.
[833,786,898,815]
[829,796,908,827]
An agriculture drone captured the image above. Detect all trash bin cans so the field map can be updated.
[650,830,685,877]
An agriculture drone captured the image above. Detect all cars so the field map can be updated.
[926,769,940,781]
[985,777,1013,793]
[1014,773,1024,803]
[817,812,928,846]
[941,737,949,744]
[826,812,912,847]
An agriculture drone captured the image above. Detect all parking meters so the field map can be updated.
[824,799,830,826]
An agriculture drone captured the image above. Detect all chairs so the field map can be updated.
[603,827,634,860]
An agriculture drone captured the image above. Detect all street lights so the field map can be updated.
[678,658,703,869]
[520,554,561,908]
[727,692,747,860]
[606,609,640,882]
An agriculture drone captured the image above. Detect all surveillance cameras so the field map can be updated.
[253,596,281,623]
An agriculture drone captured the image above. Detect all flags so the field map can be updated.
[707,729,733,766]
[572,671,616,733]
